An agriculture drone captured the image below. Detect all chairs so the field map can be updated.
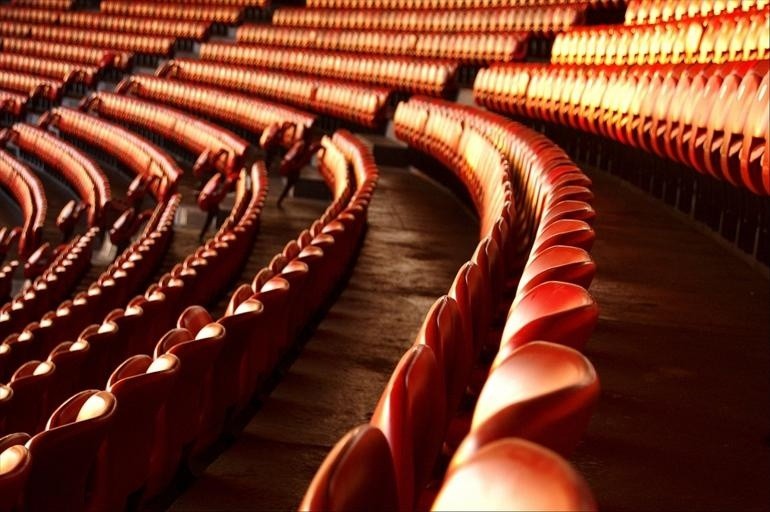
[0,0,770,512]
